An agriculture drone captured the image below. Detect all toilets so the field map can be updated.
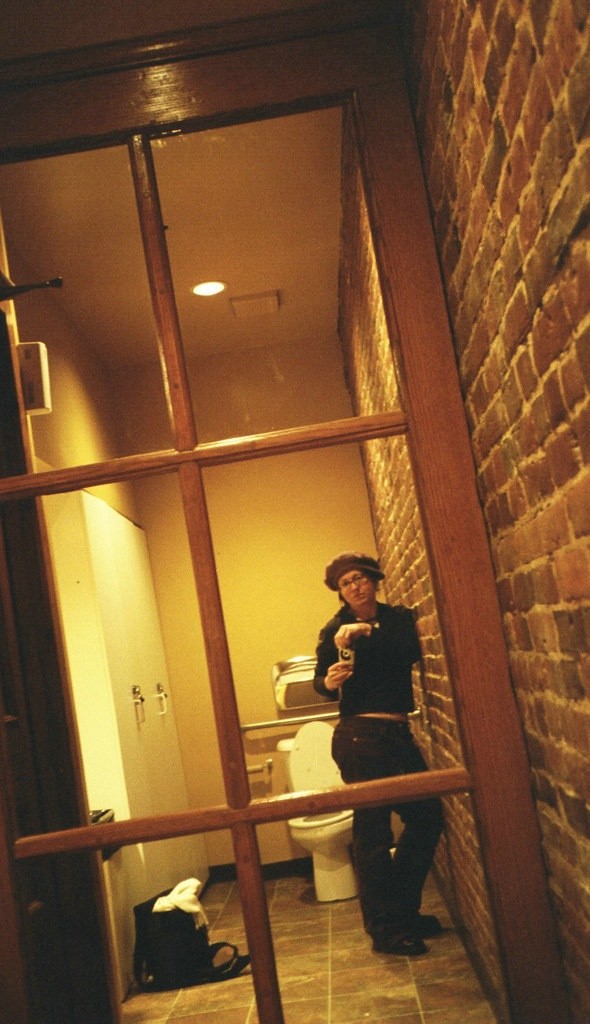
[285,720,359,903]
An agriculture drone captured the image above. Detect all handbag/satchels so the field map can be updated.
[132,889,251,991]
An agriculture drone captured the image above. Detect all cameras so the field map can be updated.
[339,647,355,667]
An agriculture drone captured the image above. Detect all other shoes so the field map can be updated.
[394,912,441,939]
[373,929,426,955]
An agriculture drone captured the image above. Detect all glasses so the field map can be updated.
[338,573,369,590]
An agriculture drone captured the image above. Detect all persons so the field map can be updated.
[313,552,444,954]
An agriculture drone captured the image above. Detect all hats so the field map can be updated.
[324,552,387,591]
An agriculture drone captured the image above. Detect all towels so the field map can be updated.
[152,877,209,931]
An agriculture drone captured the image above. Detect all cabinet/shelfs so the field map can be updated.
[82,488,211,894]
